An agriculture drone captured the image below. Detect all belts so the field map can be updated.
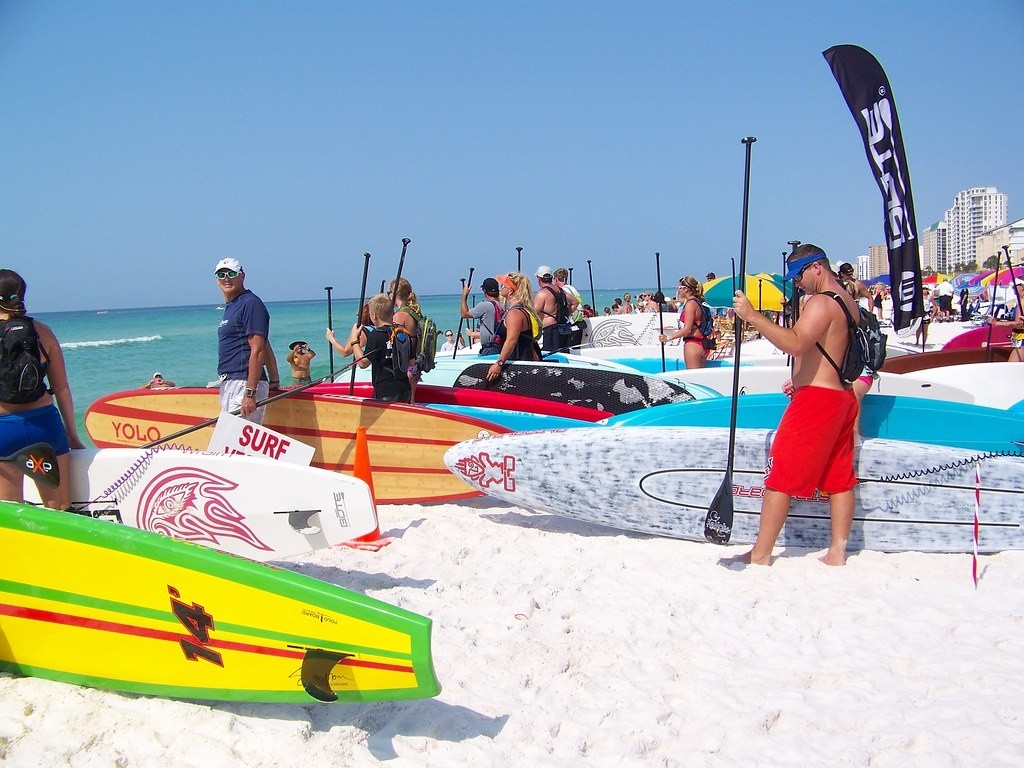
[482,343,500,348]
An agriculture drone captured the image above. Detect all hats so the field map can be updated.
[781,252,826,282]
[838,263,854,273]
[214,258,242,274]
[496,273,518,291]
[922,285,928,288]
[673,297,676,299]
[706,273,715,277]
[535,265,554,278]
[480,278,499,292]
[154,372,161,378]
[289,342,307,350]
[639,290,651,298]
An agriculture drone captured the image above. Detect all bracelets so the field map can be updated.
[270,379,280,383]
[350,341,360,346]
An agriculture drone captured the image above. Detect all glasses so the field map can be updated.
[793,263,822,282]
[846,273,853,276]
[446,334,453,337]
[707,278,713,280]
[678,285,687,289]
[215,271,242,279]
[641,295,650,297]
[922,288,928,290]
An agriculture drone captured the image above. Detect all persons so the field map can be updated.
[929,284,941,323]
[287,341,316,385]
[139,372,176,388]
[439,264,717,381]
[326,278,424,402]
[720,243,891,567]
[935,276,955,322]
[988,278,1024,361]
[0,269,87,515]
[212,257,280,425]
[779,292,814,329]
[914,285,938,347]
[957,288,969,321]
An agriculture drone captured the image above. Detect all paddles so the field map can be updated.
[985,251,1002,363]
[703,137,756,543]
[655,251,665,372]
[459,278,471,349]
[138,346,382,448]
[472,296,475,344]
[453,268,474,359]
[587,260,595,317]
[568,268,573,285]
[516,247,523,272]
[324,287,333,383]
[349,253,372,396]
[731,258,736,339]
[392,238,411,310]
[1002,245,1024,316]
[757,241,802,367]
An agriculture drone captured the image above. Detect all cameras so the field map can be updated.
[298,345,303,348]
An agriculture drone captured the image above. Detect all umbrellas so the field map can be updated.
[920,266,1023,320]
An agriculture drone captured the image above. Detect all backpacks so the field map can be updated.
[385,307,437,380]
[479,300,509,344]
[542,286,583,337]
[0,314,50,403]
[803,290,888,386]
[683,298,714,336]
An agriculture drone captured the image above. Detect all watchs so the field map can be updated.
[245,387,256,399]
[307,347,310,351]
[667,336,669,342]
[498,358,506,366]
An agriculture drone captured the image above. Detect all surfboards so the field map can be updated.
[333,325,1024,457]
[0,448,443,704]
[84,388,515,504]
[442,427,1021,552]
